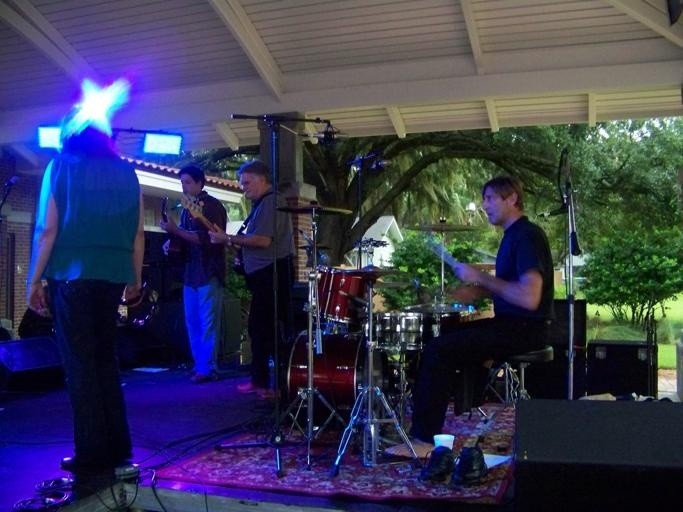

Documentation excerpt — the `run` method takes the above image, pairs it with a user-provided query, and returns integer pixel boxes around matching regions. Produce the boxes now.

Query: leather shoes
[61,455,77,470]
[235,382,280,399]
[185,370,217,384]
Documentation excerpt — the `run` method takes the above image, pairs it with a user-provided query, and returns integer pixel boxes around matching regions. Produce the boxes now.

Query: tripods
[216,106,321,477]
[288,268,421,475]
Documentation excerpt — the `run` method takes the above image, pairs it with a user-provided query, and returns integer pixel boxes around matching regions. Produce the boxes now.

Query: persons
[25,109,145,475]
[208,161,296,399]
[159,164,226,381]
[384,176,554,459]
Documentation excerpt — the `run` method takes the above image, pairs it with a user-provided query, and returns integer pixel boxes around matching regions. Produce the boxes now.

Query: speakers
[512,399,682,512]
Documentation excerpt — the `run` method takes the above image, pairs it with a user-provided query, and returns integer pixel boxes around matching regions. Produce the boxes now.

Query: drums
[362,312,424,352]
[287,331,383,428]
[313,265,368,326]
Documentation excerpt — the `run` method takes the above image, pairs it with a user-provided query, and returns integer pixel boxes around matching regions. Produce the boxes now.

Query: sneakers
[418,447,488,485]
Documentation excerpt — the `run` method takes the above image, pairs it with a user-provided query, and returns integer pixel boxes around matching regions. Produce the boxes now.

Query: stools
[504,344,555,408]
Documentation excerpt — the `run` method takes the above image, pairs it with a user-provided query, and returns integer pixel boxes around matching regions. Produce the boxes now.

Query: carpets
[148,401,518,507]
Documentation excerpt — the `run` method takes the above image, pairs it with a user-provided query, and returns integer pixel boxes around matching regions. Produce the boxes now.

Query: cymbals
[331,266,410,284]
[404,304,469,315]
[275,204,354,215]
[297,246,332,249]
[406,222,481,232]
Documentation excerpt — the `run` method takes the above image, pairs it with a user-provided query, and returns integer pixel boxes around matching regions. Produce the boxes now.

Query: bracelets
[25,280,42,285]
[224,234,233,246]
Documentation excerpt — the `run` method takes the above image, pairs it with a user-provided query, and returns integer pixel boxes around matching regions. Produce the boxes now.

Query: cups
[433,433,456,452]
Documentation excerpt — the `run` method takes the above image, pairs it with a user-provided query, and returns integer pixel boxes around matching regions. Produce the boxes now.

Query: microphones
[540,203,583,217]
[299,231,330,264]
[298,132,348,140]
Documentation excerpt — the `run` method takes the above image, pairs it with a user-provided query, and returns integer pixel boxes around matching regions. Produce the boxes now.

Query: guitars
[181,193,244,275]
[161,195,184,273]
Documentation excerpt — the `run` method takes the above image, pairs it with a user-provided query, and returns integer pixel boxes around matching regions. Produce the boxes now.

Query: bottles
[267,355,275,388]
